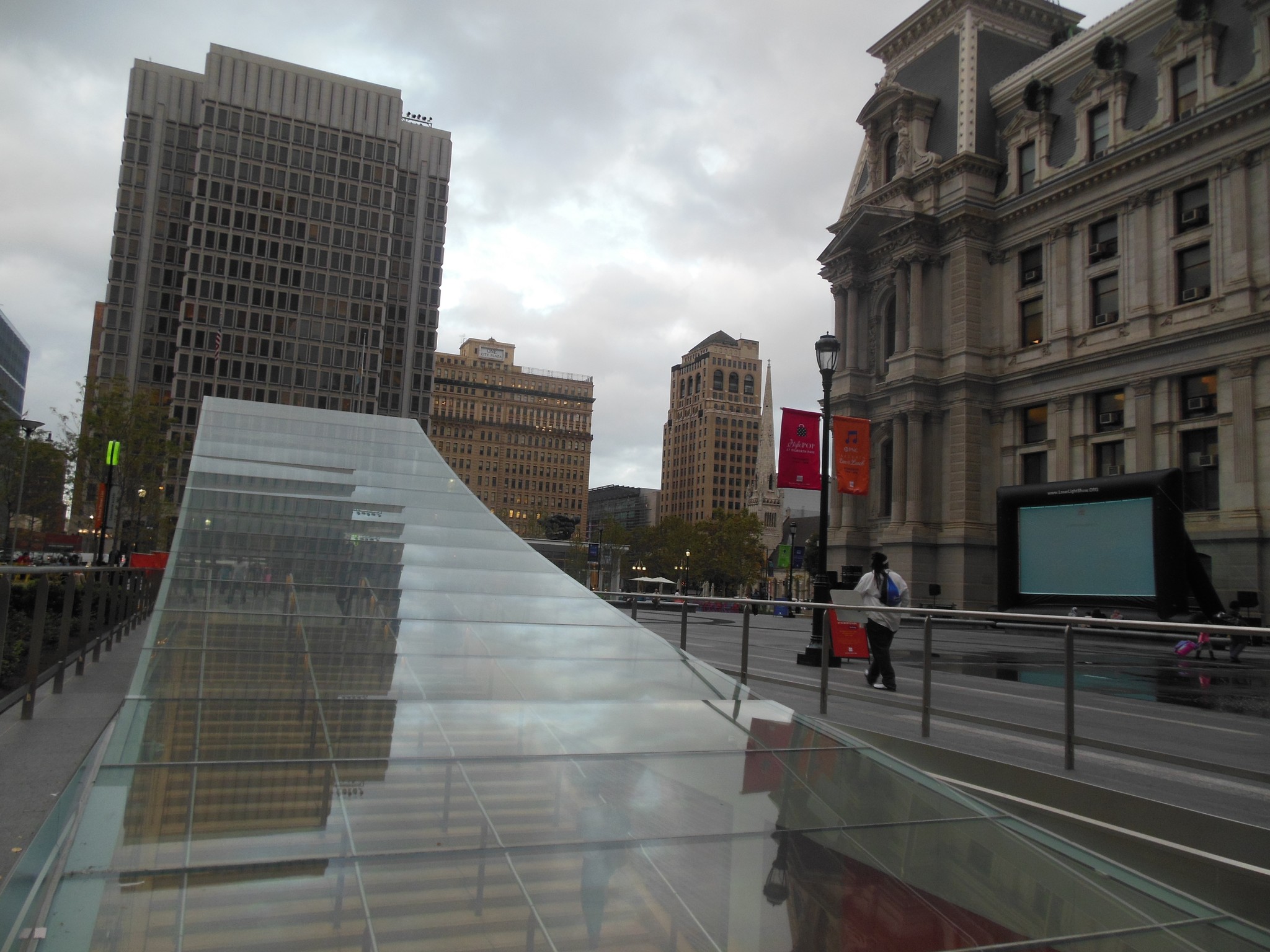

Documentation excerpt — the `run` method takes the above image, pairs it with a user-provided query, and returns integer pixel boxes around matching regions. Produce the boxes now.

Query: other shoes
[864,669,872,685]
[873,683,891,691]
[1231,658,1240,663]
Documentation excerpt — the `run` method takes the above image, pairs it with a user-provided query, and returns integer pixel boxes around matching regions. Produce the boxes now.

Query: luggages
[1173,640,1203,657]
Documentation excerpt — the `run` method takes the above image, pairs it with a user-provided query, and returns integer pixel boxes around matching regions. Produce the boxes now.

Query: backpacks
[875,571,901,606]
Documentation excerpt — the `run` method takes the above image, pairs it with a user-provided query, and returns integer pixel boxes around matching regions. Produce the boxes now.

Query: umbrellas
[629,577,653,594]
[703,581,715,598]
[646,577,678,595]
[739,584,743,599]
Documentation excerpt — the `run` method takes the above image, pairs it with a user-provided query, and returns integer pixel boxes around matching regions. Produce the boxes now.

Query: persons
[794,598,801,614]
[1067,601,1250,663]
[750,589,760,616]
[853,551,910,692]
[589,584,680,611]
[227,556,248,605]
[334,565,356,625]
[17,546,122,585]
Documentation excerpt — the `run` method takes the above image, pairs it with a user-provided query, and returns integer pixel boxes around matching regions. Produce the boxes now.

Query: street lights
[794,329,844,666]
[632,558,647,593]
[133,485,147,552]
[8,418,46,567]
[758,548,777,599]
[674,558,689,596]
[782,522,796,618]
[684,549,691,597]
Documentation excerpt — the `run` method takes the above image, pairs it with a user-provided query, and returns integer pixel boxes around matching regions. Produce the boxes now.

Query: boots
[1209,652,1218,660]
[1195,651,1202,659]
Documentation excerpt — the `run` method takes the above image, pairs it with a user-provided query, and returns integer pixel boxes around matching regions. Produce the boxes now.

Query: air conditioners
[1198,454,1218,466]
[1088,243,1106,256]
[1026,271,1041,280]
[1096,314,1114,326]
[1100,413,1118,424]
[1182,209,1204,223]
[1108,465,1124,476]
[1183,288,1205,301]
[1188,397,1209,410]
